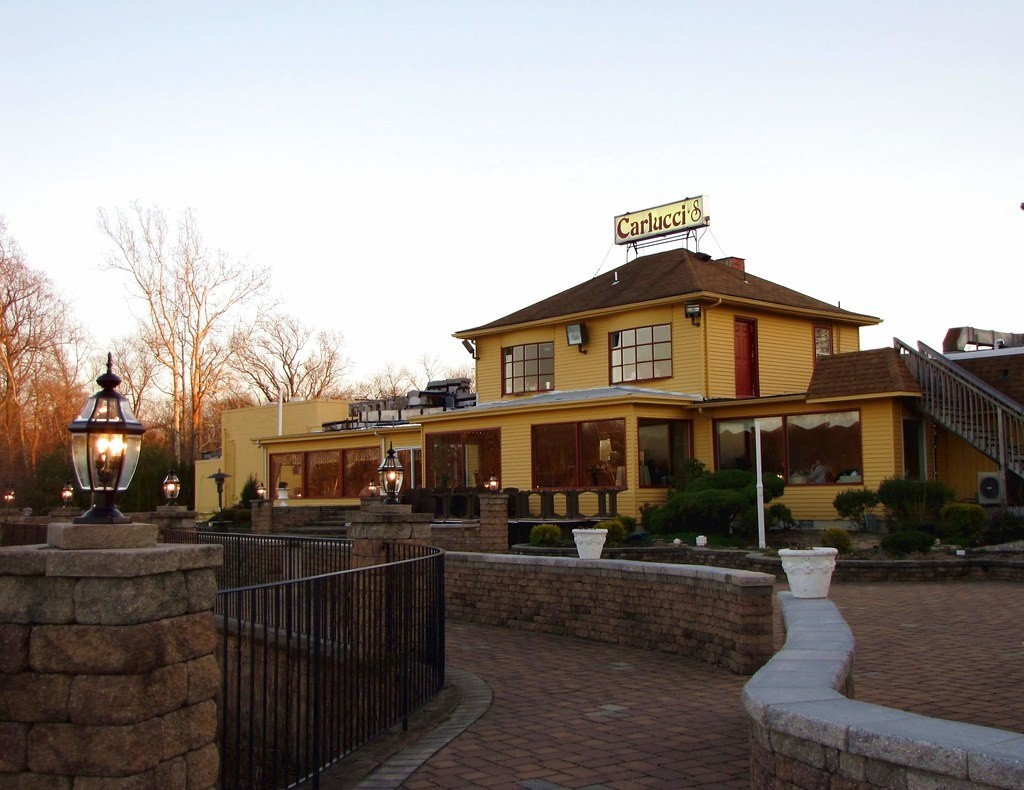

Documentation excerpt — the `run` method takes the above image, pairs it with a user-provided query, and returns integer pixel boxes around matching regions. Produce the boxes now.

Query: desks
[790,476,812,483]
[839,474,861,482]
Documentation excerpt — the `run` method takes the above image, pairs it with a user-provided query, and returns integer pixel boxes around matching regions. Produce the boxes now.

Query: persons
[735,456,747,470]
[810,459,826,484]
[835,468,862,482]
[823,462,834,480]
[565,459,573,480]
[591,466,599,485]
[778,457,784,474]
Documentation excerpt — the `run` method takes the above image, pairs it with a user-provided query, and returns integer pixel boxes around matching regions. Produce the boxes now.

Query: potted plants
[778,544,838,599]
[276,481,289,507]
[571,527,608,560]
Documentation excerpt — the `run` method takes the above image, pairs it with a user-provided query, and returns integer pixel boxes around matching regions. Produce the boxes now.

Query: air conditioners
[978,473,1001,504]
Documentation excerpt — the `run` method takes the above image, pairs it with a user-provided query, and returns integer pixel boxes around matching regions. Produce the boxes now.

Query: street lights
[206,466,232,513]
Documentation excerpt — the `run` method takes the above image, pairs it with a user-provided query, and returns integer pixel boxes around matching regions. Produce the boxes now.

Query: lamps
[206,468,231,492]
[488,473,500,493]
[61,480,75,508]
[462,339,479,360]
[368,478,377,497]
[377,440,404,503]
[68,352,146,523]
[566,323,588,354]
[684,302,701,326]
[162,467,181,506]
